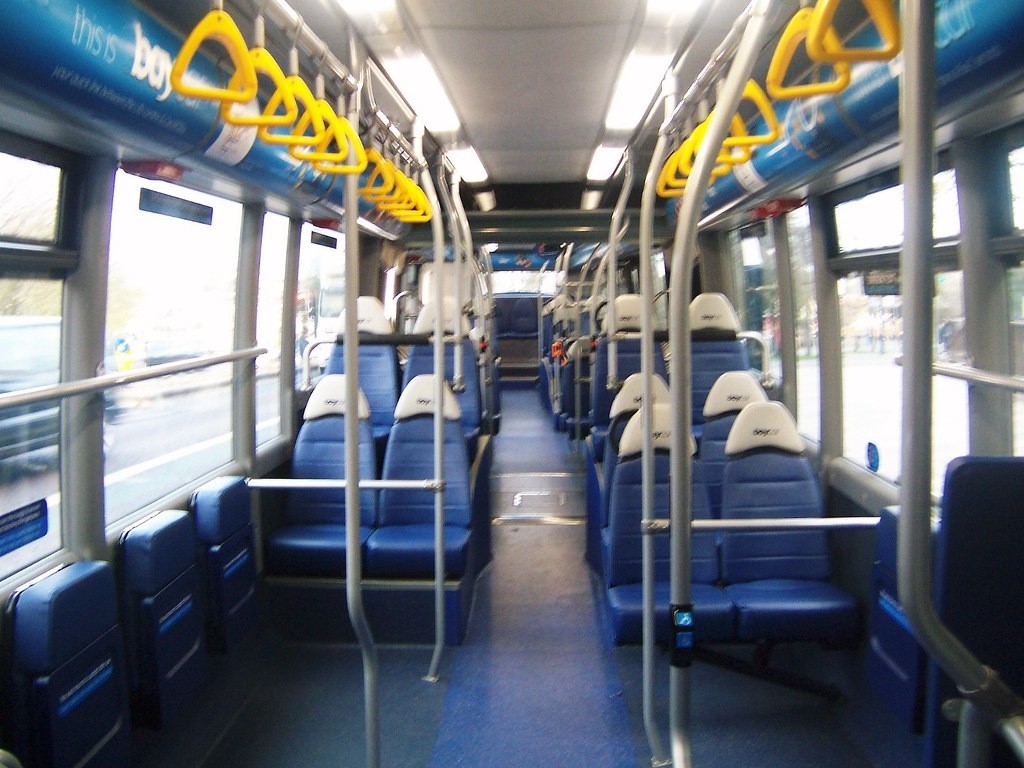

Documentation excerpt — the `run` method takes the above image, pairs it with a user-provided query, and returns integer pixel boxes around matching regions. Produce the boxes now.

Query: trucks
[316,277,345,374]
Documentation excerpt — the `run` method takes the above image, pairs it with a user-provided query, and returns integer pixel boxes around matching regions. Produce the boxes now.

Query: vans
[0,315,120,468]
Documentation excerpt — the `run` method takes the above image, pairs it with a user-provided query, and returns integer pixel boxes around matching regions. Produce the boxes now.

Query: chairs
[2,559,130,768]
[589,293,670,462]
[326,295,397,442]
[189,476,258,656]
[720,402,859,641]
[365,374,473,581]
[689,291,749,437]
[264,374,377,578]
[605,406,735,648]
[924,456,1024,768]
[700,371,769,546]
[117,510,208,731]
[606,373,696,529]
[401,296,482,445]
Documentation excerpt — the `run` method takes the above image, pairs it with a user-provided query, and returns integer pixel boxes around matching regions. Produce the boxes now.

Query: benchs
[494,298,545,341]
